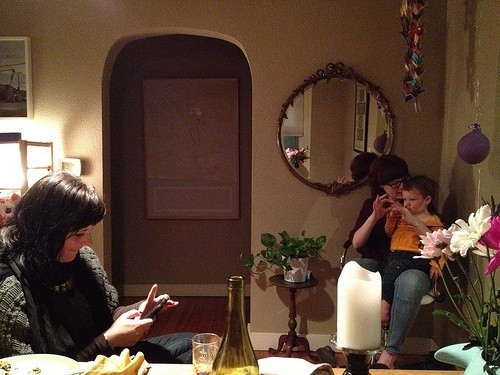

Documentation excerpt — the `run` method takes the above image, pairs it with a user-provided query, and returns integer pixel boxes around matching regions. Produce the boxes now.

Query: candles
[336,260,382,350]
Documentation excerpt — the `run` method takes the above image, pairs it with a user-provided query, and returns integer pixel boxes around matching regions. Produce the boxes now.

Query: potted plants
[240,230,327,282]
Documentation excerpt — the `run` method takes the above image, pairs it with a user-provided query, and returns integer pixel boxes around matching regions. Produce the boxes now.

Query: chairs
[340,241,444,369]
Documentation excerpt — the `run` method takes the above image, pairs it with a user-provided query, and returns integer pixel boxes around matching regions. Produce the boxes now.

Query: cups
[191,333,221,375]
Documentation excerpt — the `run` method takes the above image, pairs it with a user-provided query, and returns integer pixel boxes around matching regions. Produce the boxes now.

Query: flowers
[414,196,500,372]
[285,144,309,169]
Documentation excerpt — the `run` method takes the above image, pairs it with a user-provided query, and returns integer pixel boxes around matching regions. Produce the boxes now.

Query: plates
[0,354,80,375]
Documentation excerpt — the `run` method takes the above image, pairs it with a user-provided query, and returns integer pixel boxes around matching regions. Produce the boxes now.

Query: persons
[0,170,208,365]
[380,174,447,322]
[336,152,378,185]
[343,155,434,369]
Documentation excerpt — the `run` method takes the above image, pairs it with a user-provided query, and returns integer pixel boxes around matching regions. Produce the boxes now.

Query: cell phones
[145,298,166,318]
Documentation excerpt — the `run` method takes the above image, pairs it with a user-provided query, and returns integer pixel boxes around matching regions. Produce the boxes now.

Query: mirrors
[277,61,396,196]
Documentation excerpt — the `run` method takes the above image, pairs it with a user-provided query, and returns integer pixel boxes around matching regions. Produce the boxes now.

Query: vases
[434,343,500,375]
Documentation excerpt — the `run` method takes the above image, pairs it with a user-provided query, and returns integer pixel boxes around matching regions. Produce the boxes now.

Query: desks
[267,275,320,361]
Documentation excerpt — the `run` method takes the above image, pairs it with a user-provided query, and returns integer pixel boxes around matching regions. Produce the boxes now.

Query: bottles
[211,275,260,375]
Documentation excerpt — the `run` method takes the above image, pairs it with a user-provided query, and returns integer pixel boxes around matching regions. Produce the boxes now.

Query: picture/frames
[0,36,34,120]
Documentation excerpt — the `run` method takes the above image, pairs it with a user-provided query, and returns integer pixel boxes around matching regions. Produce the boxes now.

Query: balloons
[374,133,387,153]
[456,127,491,166]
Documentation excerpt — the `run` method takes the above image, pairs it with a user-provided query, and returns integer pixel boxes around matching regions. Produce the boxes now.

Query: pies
[79,349,144,375]
